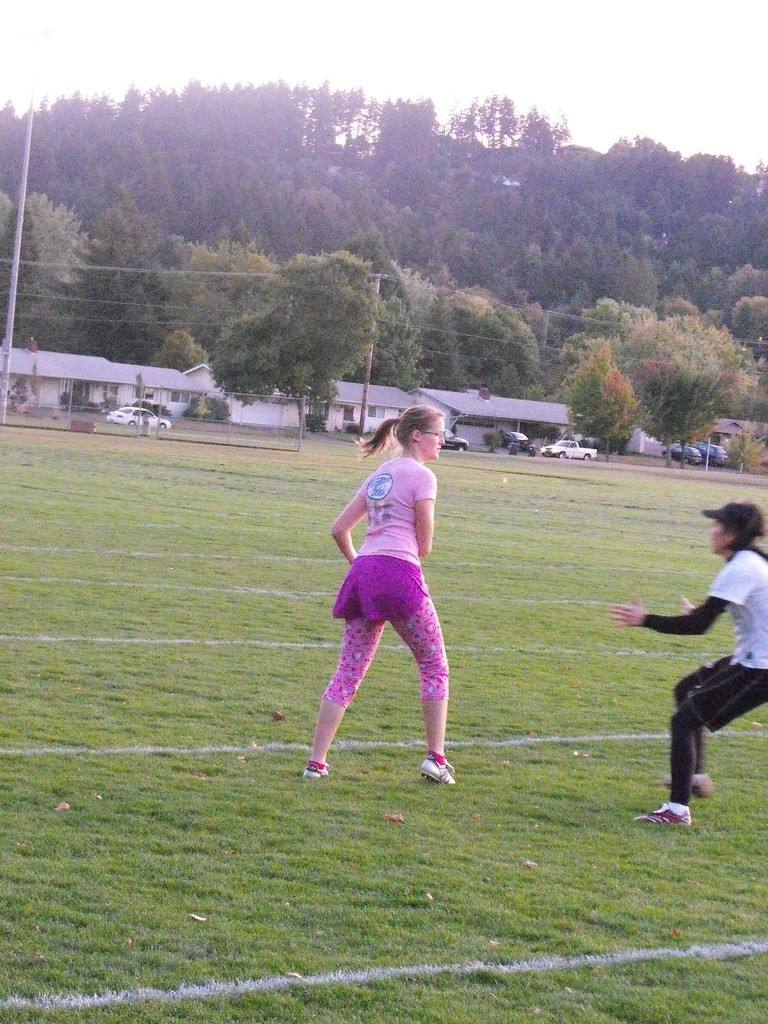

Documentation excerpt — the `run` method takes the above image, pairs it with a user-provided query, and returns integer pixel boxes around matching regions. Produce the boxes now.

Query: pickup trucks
[541,440,598,461]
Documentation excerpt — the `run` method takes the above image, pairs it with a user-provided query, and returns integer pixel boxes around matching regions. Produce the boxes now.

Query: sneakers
[632,803,694,826]
[661,773,720,798]
[417,751,456,786]
[303,763,329,779]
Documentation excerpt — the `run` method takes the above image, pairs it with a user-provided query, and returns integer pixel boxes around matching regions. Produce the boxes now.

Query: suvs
[662,447,702,465]
[503,431,531,452]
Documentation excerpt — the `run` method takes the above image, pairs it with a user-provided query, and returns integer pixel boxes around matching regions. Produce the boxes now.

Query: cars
[440,429,469,451]
[106,407,171,429]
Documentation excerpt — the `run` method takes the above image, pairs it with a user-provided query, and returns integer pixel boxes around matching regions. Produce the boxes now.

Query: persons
[607,502,768,823]
[303,406,455,785]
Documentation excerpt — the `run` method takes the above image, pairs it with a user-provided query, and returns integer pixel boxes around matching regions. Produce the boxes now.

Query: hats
[700,498,765,539]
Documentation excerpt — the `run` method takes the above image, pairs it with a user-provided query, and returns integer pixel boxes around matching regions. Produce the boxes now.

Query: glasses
[422,430,448,439]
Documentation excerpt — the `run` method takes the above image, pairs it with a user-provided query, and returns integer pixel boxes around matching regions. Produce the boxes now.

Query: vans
[696,442,728,467]
